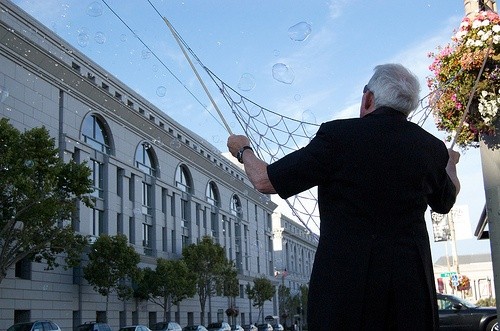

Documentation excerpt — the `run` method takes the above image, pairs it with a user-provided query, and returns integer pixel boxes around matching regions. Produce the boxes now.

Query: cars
[118,319,285,331]
[75,320,112,331]
[437,293,499,331]
[6,319,61,331]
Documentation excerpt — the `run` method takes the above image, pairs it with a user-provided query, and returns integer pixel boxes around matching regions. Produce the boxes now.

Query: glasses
[363,85,374,96]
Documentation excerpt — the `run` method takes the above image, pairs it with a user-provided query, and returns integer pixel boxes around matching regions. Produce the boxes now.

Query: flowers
[424,10,500,151]
[449,274,470,290]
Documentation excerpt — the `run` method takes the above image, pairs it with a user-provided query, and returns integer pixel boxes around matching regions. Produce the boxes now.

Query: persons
[228,63,461,331]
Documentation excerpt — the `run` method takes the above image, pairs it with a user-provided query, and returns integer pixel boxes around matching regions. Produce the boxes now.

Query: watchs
[237,146,254,164]
